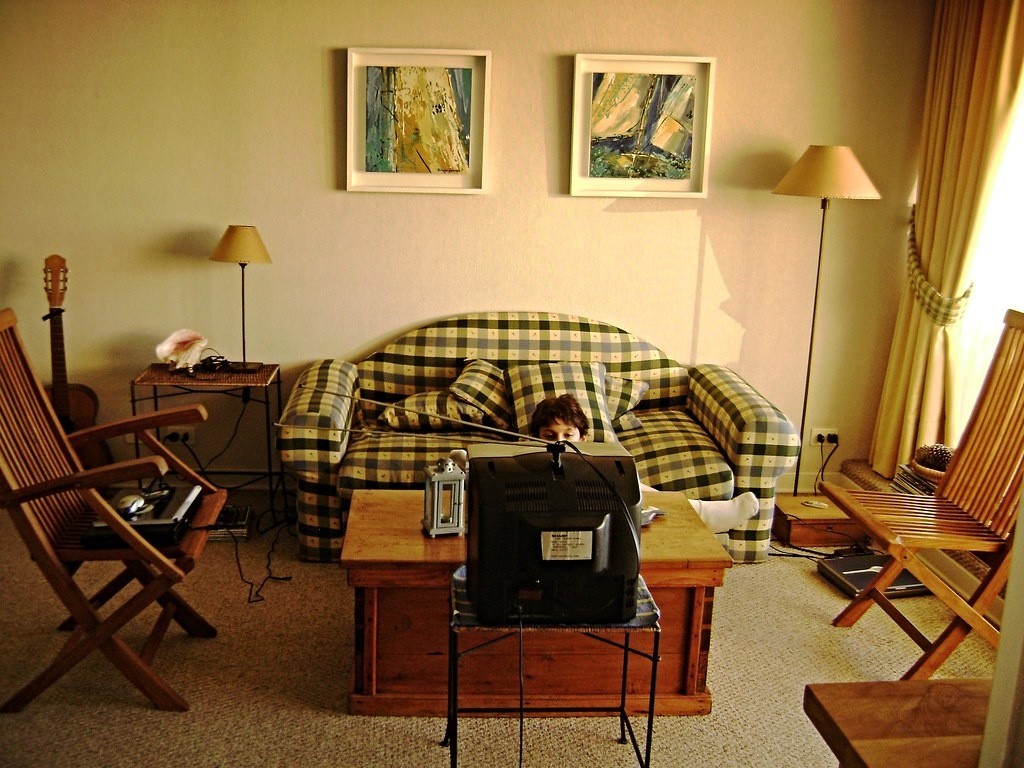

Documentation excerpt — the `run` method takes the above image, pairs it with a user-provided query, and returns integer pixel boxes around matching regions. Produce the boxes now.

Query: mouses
[117,494,144,521]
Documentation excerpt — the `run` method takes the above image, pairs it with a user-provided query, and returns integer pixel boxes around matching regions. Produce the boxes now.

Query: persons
[529,392,760,534]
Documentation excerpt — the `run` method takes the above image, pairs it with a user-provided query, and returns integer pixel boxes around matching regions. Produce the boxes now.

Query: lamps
[208,225,273,373]
[771,143,884,498]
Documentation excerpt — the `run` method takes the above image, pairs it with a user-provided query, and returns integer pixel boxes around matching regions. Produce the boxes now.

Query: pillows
[604,373,651,432]
[377,390,467,430]
[450,357,520,439]
[502,362,621,444]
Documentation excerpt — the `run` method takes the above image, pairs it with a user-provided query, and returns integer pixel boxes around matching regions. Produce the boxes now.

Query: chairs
[832,309,1024,681]
[0,308,228,715]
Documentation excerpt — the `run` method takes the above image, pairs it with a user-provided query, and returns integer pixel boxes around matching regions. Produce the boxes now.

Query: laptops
[82,484,205,550]
[817,554,933,600]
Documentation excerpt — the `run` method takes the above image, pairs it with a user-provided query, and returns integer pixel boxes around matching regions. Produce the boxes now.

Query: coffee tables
[340,489,735,716]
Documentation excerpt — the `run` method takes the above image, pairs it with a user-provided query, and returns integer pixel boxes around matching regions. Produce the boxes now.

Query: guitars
[40,254,115,499]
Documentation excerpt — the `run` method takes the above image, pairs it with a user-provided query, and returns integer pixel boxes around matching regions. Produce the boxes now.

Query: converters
[834,547,874,557]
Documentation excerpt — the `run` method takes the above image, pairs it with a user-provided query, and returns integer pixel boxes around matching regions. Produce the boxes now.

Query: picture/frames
[571,53,716,199]
[347,45,495,197]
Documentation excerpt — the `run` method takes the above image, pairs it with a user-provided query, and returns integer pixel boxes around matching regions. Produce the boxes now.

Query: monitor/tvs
[273,383,643,628]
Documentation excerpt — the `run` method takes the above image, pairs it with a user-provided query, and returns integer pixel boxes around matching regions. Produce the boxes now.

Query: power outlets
[164,425,196,446]
[810,428,839,446]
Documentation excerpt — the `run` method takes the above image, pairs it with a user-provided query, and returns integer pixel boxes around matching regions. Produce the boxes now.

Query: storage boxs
[773,492,873,545]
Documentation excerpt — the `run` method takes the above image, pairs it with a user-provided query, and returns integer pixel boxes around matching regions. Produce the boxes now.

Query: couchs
[276,311,802,566]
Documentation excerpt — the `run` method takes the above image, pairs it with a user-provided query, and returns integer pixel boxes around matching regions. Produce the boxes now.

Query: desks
[442,566,662,768]
[130,361,283,533]
[803,677,1024,767]
